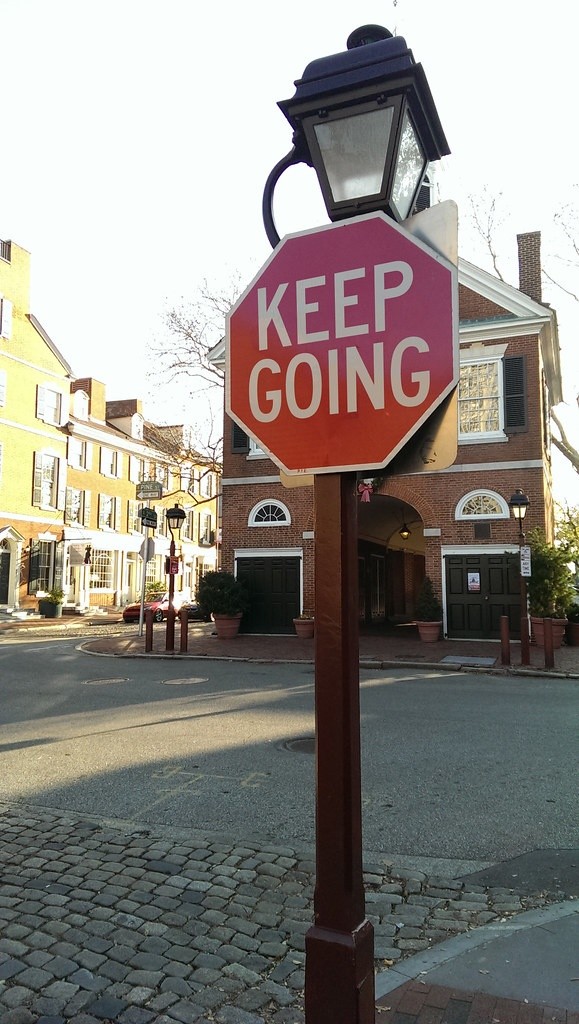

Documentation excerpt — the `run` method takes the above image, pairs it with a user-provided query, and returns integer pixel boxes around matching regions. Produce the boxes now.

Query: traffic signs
[136,481,162,500]
[140,506,157,528]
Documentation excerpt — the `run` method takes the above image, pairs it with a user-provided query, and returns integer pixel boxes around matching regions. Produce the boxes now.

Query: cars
[122,592,182,623]
[178,598,215,623]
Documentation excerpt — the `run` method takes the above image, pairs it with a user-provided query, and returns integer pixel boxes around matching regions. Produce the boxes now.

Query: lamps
[399,508,411,540]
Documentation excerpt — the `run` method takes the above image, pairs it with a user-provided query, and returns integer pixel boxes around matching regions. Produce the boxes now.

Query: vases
[293,616,314,639]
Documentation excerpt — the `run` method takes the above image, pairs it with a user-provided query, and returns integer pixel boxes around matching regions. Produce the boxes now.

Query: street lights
[508,488,531,665]
[263,22,450,1024]
[165,503,187,650]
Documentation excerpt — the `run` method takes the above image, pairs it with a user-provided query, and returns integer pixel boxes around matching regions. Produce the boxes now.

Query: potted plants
[195,569,245,641]
[504,526,578,651]
[38,586,64,618]
[565,601,579,646]
[415,576,443,643]
